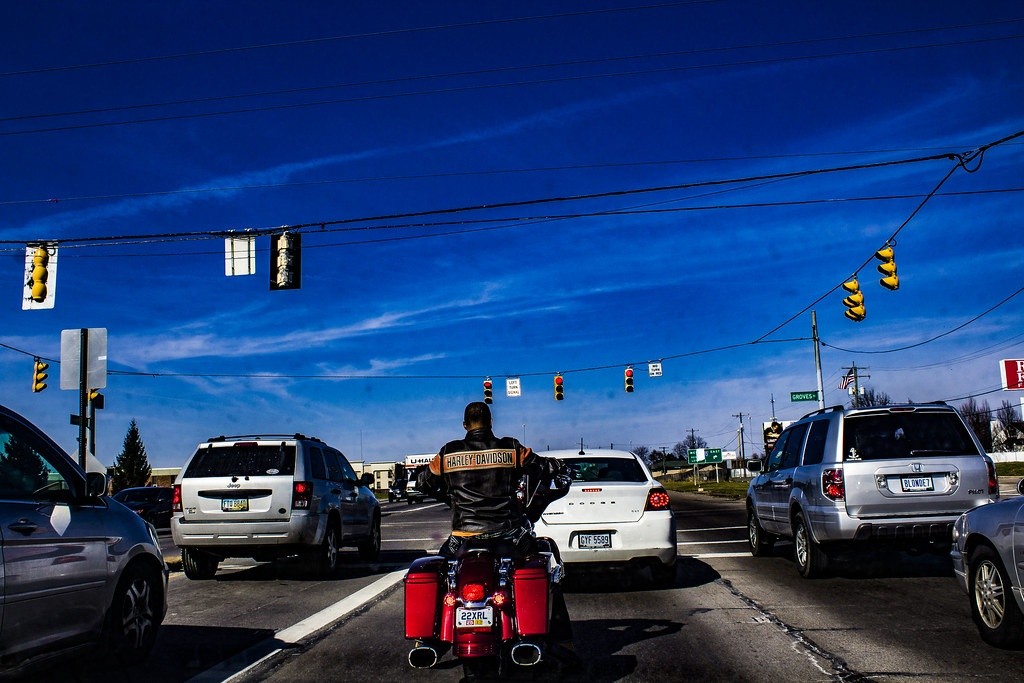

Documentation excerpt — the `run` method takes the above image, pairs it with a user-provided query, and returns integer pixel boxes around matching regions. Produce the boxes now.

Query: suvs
[746,401,1003,580]
[169,432,382,580]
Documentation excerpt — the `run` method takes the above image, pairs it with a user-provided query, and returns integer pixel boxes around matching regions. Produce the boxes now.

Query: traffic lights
[483,380,493,405]
[553,376,564,400]
[876,246,900,291]
[842,279,866,322]
[625,368,634,393]
[88,388,100,401]
[31,361,49,393]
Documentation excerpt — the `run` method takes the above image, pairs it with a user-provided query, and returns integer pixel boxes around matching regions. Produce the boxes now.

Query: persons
[416,401,550,562]
[764,422,783,443]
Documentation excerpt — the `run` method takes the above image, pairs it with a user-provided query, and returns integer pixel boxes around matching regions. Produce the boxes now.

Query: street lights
[521,424,526,446]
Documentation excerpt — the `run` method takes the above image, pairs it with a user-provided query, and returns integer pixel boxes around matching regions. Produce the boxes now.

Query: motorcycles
[402,536,554,683]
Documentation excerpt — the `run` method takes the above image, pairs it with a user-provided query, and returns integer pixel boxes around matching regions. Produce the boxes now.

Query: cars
[950,478,1024,650]
[388,479,407,505]
[109,487,174,529]
[0,404,170,683]
[533,448,677,589]
[405,473,428,505]
